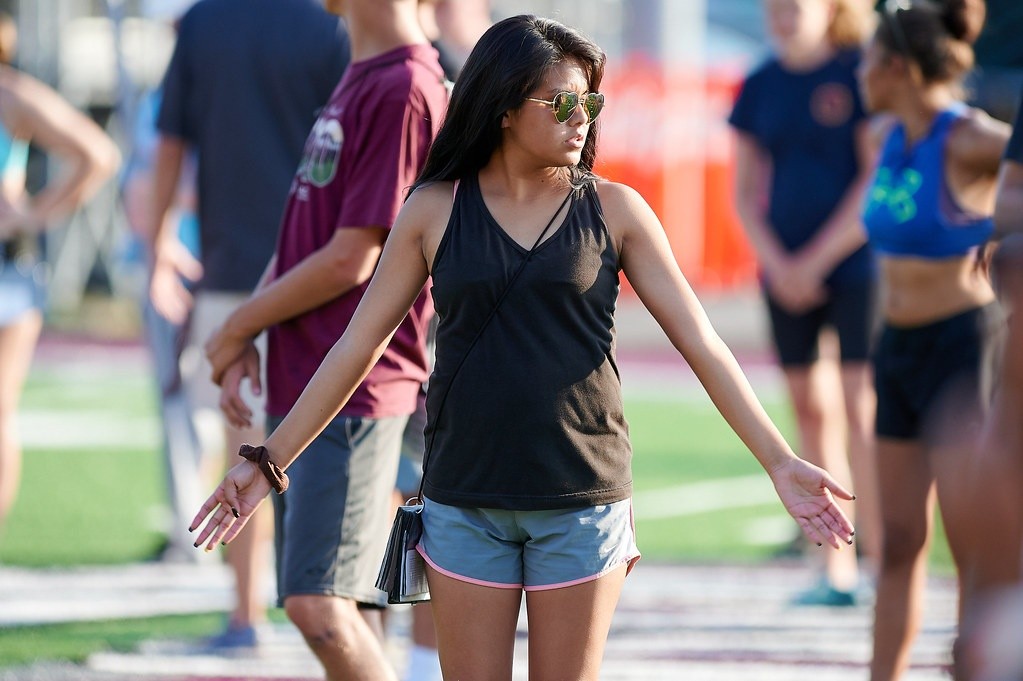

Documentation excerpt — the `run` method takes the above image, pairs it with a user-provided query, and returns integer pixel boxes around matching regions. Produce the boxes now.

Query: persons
[767,0,1023,681]
[724,0,880,602]
[142,0,351,652]
[189,15,856,681]
[204,0,451,681]
[0,0,503,657]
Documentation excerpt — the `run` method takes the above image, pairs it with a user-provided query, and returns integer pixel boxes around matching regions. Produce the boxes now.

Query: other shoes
[194,623,276,658]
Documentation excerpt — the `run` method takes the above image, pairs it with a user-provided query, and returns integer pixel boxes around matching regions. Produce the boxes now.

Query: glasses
[874,0,909,49]
[522,91,604,125]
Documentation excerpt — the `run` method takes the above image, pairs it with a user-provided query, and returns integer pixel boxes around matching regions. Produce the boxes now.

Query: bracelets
[237,441,290,495]
